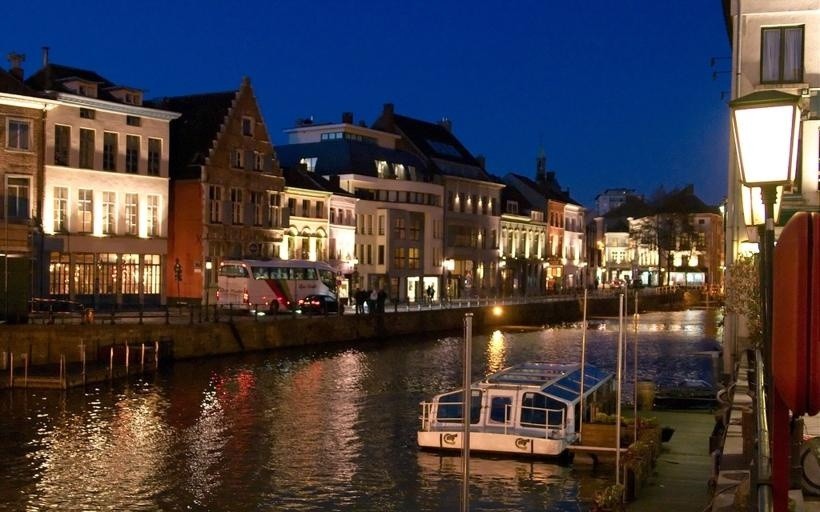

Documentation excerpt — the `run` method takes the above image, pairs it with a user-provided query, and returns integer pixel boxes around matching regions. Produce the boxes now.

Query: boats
[416,358,618,462]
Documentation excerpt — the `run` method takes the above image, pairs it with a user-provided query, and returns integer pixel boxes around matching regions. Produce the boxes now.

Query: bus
[218,260,341,314]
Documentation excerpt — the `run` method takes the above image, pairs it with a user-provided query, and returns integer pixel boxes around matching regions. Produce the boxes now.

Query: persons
[427,284,431,297]
[429,285,435,300]
[594,278,599,290]
[353,285,388,315]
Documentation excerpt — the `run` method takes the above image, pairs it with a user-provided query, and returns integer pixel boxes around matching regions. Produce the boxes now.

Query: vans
[25,298,86,326]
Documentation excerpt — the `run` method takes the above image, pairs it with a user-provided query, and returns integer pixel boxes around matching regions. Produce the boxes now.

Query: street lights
[443,258,455,305]
[737,178,784,345]
[203,255,211,322]
[725,91,803,510]
[720,265,726,278]
[600,266,608,294]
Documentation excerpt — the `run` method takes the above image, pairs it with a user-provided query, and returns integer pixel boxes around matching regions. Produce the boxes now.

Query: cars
[302,294,345,313]
